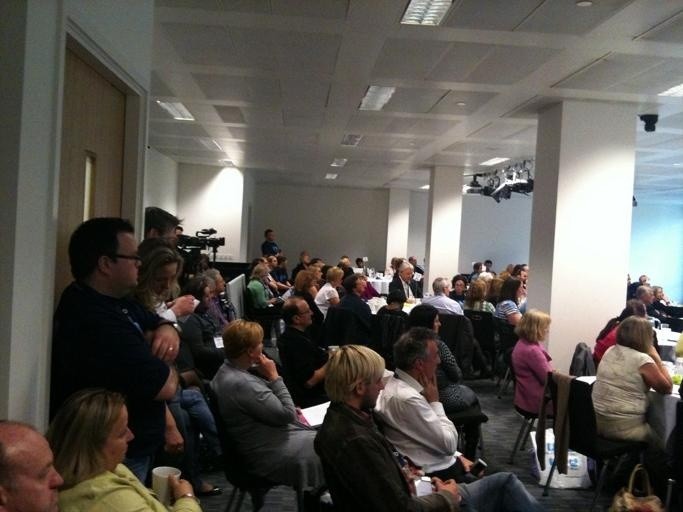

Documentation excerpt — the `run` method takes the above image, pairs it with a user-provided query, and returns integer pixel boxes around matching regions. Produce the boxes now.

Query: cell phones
[469,458,488,476]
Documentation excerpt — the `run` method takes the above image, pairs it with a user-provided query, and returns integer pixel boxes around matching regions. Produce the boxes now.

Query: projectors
[463,184,484,196]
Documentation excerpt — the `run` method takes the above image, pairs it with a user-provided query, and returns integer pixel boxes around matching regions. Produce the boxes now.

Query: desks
[646,380,679,476]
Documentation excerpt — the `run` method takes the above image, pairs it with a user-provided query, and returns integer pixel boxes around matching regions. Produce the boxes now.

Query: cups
[151,465,180,502]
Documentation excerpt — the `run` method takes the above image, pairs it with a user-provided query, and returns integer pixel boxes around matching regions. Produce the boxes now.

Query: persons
[592,274,682,511]
[1,205,547,512]
[510,309,556,418]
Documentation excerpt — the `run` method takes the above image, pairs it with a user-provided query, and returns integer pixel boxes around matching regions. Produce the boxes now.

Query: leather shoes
[196,485,224,497]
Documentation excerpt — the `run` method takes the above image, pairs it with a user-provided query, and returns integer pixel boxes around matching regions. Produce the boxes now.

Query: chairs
[542,370,648,509]
[505,348,556,467]
[570,343,595,374]
[628,281,682,357]
[211,265,505,504]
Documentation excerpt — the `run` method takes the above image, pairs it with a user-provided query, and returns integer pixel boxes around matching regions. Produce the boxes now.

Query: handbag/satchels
[606,463,667,512]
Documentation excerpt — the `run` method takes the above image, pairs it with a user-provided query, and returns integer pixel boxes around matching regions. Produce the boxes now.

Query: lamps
[459,159,536,203]
